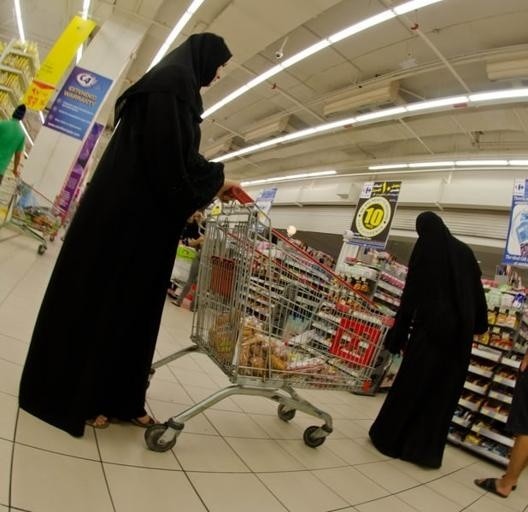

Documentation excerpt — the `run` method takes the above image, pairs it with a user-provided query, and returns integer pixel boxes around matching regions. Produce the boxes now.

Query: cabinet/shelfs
[447,282,528,470]
[0,38,39,122]
[319,243,407,396]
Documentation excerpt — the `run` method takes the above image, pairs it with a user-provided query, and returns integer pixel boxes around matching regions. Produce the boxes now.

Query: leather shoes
[167,291,197,313]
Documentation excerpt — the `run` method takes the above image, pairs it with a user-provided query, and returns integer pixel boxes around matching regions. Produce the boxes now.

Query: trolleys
[139,186,392,458]
[0,176,68,256]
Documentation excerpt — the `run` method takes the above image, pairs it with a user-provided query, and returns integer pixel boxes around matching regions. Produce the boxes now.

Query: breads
[239,328,288,378]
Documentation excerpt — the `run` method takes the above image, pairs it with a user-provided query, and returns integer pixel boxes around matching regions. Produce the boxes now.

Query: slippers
[474,477,511,498]
[501,473,517,491]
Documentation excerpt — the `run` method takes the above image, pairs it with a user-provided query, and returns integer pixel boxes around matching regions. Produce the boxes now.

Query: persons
[471,346,528,500]
[169,209,212,311]
[1,103,27,180]
[16,33,244,438]
[366,212,488,470]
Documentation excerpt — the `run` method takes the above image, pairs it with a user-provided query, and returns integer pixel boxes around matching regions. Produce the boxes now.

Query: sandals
[82,410,110,429]
[129,411,159,428]
